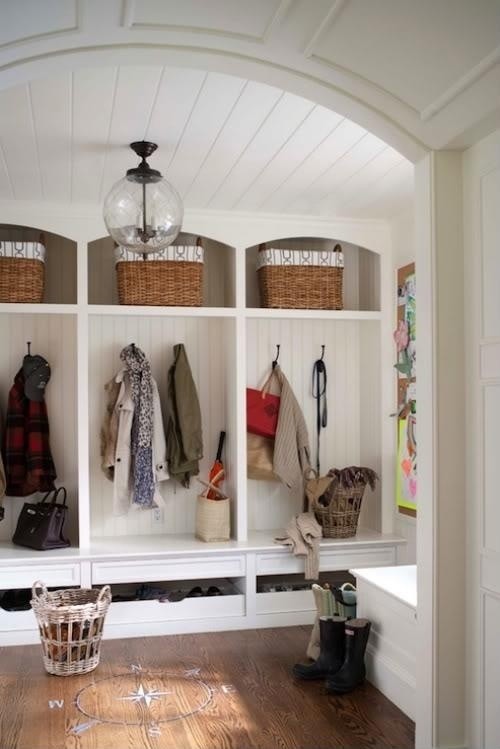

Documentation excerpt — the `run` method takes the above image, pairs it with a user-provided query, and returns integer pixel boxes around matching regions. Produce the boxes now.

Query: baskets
[258,239,345,310]
[0,232,48,303]
[303,466,366,539]
[112,237,205,309]
[32,579,114,677]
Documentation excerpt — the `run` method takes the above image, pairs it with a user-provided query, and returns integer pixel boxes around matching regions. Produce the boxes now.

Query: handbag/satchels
[306,581,358,661]
[193,470,233,544]
[247,368,284,481]
[12,487,72,551]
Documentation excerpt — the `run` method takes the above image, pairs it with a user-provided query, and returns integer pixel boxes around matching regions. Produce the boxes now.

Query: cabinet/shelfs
[0,201,414,649]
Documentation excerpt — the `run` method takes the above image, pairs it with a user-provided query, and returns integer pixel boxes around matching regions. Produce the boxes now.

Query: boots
[292,615,348,680]
[321,617,371,695]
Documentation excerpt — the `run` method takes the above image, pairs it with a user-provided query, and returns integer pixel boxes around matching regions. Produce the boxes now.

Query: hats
[20,353,52,402]
